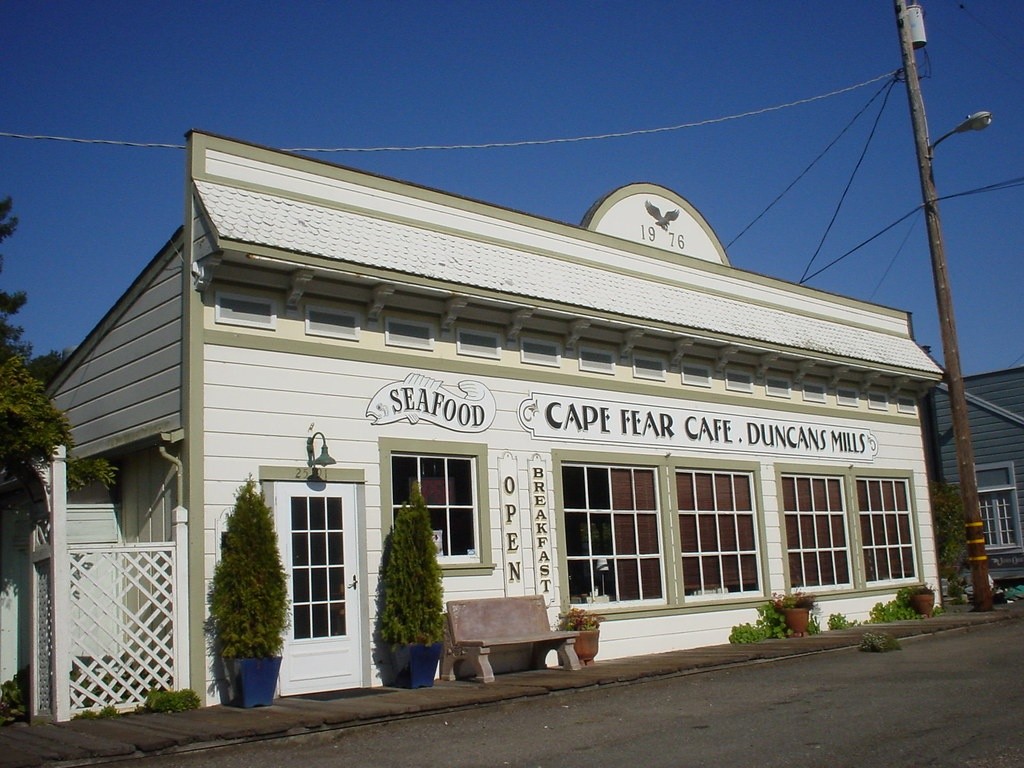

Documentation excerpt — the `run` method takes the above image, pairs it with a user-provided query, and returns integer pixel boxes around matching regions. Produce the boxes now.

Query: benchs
[444,595,582,683]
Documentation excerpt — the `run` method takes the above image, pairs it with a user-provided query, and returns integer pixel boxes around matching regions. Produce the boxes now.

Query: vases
[785,608,809,632]
[574,631,600,661]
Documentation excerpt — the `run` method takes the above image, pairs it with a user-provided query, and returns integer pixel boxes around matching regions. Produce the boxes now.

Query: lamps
[307,431,337,467]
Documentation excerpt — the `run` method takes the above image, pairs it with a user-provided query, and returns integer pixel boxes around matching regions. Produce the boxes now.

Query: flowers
[554,607,606,632]
[770,587,817,609]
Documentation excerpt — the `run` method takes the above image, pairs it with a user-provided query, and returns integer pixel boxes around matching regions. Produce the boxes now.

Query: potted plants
[201,470,292,709]
[380,479,447,688]
[943,545,967,573]
[911,584,939,613]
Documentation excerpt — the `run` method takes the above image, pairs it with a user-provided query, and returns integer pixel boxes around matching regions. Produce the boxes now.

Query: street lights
[904,112,994,612]
